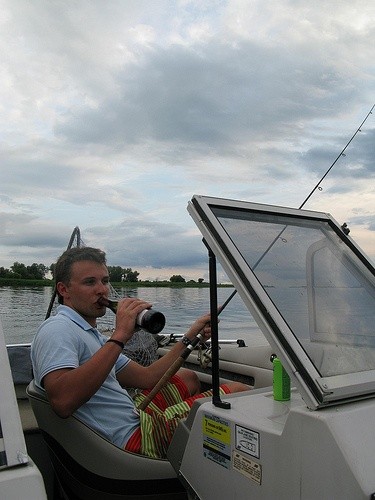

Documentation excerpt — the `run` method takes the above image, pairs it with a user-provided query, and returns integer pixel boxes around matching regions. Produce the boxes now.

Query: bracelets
[105,339,125,350]
[181,335,191,345]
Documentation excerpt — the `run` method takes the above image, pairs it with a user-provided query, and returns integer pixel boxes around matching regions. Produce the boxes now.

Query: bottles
[97,296,166,336]
[273,355,291,402]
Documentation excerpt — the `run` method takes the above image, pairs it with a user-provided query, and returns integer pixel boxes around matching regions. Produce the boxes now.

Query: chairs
[23,375,180,480]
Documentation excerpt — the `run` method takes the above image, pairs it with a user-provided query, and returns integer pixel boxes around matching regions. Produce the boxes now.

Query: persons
[30,247,254,459]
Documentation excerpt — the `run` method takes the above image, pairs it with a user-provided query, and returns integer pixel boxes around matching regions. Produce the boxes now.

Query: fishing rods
[136,103,375,412]
